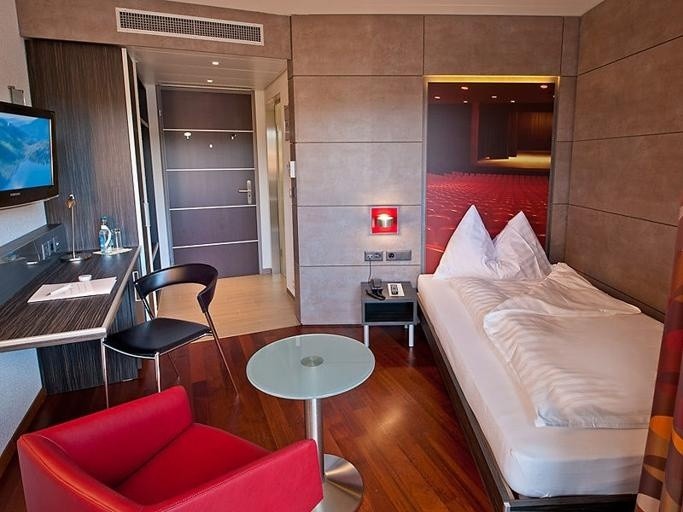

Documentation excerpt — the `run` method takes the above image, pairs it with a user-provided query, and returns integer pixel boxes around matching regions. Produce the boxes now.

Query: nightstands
[361,281,417,348]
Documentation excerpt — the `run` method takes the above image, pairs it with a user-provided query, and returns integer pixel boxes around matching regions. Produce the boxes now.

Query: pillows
[432,203,552,284]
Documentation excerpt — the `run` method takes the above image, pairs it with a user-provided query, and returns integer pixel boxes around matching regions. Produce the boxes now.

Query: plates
[94,249,131,255]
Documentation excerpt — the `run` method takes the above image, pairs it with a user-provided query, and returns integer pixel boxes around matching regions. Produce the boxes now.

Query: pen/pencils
[46,287,64,296]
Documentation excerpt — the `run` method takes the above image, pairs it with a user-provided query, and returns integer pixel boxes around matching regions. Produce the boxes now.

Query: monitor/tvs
[0,101,61,210]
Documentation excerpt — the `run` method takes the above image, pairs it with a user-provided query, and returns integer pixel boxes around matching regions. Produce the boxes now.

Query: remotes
[391,284,399,295]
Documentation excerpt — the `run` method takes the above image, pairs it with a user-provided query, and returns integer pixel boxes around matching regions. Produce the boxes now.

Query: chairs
[100,262,238,409]
[15,383,323,512]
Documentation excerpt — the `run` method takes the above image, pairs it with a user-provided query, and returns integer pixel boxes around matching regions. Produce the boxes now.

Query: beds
[418,263,665,511]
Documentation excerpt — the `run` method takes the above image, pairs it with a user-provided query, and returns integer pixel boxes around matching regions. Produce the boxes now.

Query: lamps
[60,195,92,263]
[370,206,398,234]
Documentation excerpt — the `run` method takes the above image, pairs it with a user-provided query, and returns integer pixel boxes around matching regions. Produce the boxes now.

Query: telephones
[371,278,383,291]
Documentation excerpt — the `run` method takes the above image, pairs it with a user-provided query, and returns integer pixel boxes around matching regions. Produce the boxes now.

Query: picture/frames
[421,74,562,273]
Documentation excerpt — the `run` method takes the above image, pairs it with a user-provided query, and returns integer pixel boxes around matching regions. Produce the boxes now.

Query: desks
[1,245,140,409]
[243,333,377,512]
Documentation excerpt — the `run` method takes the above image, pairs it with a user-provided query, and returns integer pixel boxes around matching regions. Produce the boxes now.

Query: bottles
[110,228,123,250]
[99,213,112,252]
